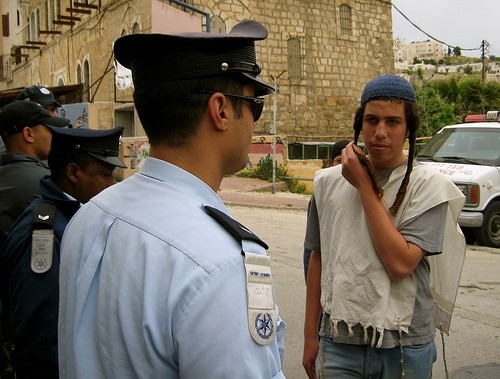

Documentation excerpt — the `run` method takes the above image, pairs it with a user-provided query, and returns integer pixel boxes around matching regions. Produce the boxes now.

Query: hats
[0,100,70,134]
[16,84,61,108]
[46,122,128,170]
[360,73,417,104]
[113,19,276,97]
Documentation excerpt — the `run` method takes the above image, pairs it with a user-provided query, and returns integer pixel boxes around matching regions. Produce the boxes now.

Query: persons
[0,100,71,251]
[57,21,287,379]
[16,86,62,116]
[304,140,351,285]
[0,126,126,379]
[302,75,467,379]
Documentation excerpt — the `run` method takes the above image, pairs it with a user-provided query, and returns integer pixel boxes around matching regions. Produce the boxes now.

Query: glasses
[204,89,265,122]
[333,156,342,166]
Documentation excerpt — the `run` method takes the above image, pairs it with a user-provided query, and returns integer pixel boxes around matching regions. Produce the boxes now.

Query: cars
[412,111,500,247]
